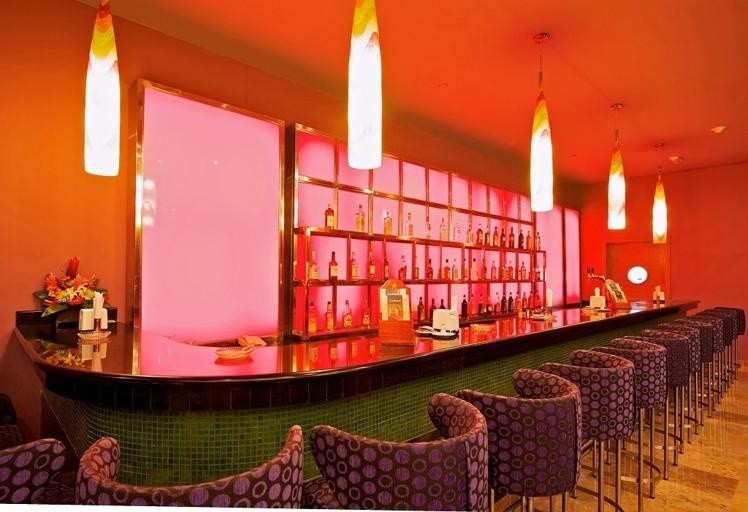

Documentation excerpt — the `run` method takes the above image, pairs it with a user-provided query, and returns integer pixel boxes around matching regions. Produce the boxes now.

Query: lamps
[527,28,670,246]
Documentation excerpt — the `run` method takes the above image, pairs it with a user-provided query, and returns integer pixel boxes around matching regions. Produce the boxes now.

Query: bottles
[95,318,101,332]
[405,212,414,236]
[383,210,392,235]
[308,250,319,279]
[326,203,335,229]
[467,223,543,251]
[438,216,448,239]
[329,250,338,279]
[367,254,542,282]
[350,251,360,279]
[356,204,365,232]
[657,295,660,306]
[308,300,371,330]
[416,291,543,324]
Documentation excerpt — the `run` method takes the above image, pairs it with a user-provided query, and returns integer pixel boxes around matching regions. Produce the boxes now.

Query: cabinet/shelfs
[287,120,537,343]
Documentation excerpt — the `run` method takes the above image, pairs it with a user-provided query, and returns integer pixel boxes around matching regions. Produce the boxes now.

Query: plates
[76,331,111,340]
[416,330,438,336]
[214,347,253,359]
[432,332,454,338]
[529,314,547,320]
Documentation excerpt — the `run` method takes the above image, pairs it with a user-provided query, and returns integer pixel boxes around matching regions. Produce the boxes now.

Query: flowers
[31,255,112,318]
